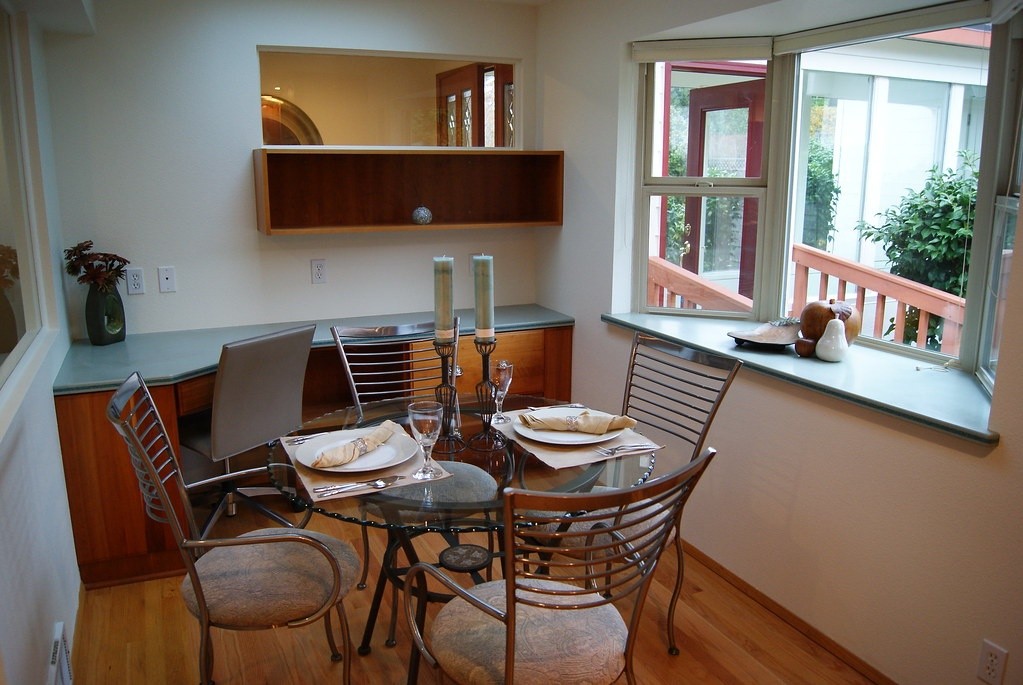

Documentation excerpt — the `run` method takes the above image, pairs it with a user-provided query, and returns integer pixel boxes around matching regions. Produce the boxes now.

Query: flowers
[56,238,132,290]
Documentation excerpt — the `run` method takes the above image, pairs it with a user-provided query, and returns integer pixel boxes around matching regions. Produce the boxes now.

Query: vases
[87,285,128,347]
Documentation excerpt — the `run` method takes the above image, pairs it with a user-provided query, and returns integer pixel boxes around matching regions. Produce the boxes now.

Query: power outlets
[125,268,148,297]
[311,259,325,284]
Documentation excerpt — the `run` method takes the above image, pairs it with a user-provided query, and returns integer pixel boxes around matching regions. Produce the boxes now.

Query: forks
[595,445,657,457]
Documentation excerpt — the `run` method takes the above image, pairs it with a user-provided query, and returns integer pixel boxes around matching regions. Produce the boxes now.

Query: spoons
[318,476,399,498]
[528,406,586,411]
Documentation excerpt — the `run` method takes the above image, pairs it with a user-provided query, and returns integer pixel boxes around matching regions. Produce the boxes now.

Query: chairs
[107,372,361,685]
[332,320,497,653]
[518,331,745,646]
[179,326,313,552]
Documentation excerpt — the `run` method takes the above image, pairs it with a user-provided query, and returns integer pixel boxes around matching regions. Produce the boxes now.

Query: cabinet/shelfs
[61,302,576,593]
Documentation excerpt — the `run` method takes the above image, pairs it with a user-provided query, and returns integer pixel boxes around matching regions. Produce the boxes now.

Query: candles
[433,254,456,344]
[471,253,496,343]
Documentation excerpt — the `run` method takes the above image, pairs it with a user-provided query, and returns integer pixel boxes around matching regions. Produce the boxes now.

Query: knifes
[313,476,406,493]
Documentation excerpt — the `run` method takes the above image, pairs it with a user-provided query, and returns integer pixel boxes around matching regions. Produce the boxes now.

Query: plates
[513,408,625,445]
[295,428,419,472]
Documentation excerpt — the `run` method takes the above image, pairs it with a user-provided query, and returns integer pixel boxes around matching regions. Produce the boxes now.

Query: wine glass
[489,360,513,424]
[408,401,443,480]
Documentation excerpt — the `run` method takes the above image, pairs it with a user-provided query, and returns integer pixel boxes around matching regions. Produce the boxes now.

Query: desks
[397,449,715,685]
[270,392,602,677]
